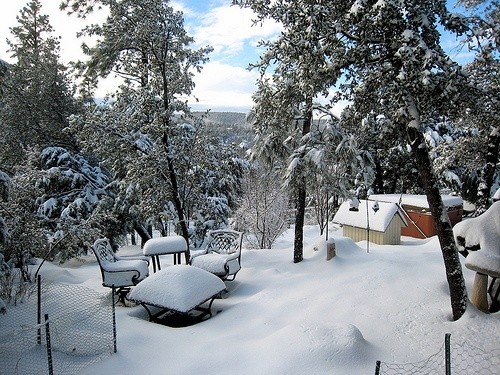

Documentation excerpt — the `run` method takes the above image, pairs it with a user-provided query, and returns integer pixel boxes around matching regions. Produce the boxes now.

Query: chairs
[189,229,245,282]
[89,236,150,307]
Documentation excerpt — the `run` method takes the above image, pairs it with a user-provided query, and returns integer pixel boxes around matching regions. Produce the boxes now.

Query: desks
[457,231,499,313]
[125,287,227,327]
[145,245,188,274]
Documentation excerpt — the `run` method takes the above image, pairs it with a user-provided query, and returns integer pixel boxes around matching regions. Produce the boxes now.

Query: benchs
[465,260,500,314]
